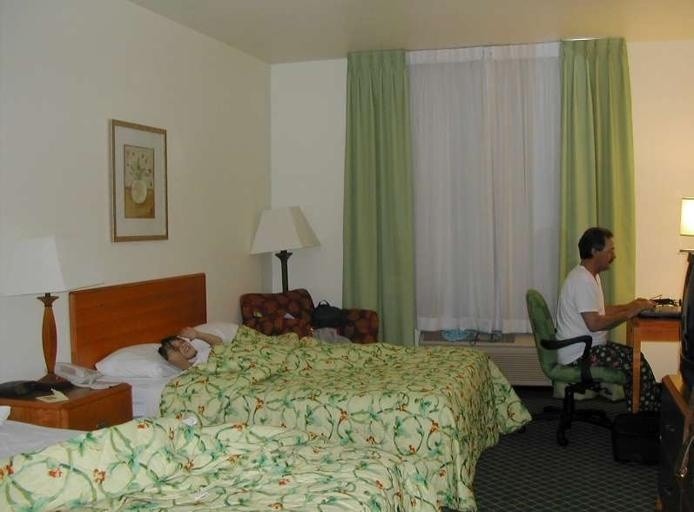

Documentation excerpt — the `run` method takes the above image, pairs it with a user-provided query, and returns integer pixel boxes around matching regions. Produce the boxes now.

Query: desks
[656,375,694,512]
[627,318,680,414]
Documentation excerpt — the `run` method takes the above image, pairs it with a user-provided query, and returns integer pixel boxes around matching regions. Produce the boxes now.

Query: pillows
[1,405,11,424]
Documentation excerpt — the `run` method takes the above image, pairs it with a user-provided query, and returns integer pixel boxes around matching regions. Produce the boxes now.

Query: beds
[0,417,456,512]
[69,273,534,511]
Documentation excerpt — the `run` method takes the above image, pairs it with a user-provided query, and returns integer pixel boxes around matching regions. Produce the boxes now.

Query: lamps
[679,197,694,237]
[5,235,105,390]
[250,206,321,292]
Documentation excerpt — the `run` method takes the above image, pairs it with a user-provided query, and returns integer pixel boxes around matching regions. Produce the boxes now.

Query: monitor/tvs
[679,254,694,386]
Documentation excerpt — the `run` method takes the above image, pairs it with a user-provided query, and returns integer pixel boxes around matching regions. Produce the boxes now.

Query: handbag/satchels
[310,299,345,330]
[610,410,661,465]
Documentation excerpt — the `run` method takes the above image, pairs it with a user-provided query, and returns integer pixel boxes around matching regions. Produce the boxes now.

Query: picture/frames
[111,119,169,241]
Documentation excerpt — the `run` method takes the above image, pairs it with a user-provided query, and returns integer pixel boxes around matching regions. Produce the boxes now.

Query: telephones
[54,362,101,384]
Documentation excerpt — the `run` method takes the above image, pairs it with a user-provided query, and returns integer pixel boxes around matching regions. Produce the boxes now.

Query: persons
[555,228,662,413]
[159,325,224,373]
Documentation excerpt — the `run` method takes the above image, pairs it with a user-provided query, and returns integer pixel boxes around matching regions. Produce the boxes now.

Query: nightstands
[1,383,133,431]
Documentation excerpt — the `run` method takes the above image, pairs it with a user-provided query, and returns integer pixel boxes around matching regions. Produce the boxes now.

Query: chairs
[241,289,378,343]
[518,290,625,447]
